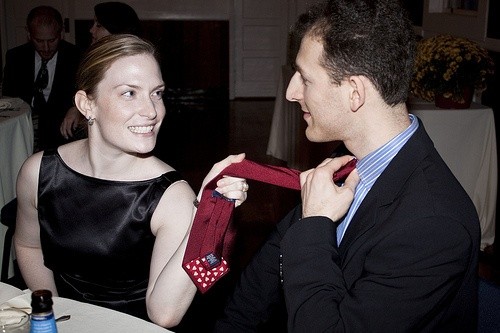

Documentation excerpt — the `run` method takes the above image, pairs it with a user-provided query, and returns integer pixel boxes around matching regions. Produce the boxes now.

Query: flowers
[408,33,494,103]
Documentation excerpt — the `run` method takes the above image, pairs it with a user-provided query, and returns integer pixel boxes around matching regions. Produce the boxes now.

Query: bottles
[29,289,58,332]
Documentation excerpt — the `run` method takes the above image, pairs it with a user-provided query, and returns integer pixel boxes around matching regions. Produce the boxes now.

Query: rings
[244,183,248,191]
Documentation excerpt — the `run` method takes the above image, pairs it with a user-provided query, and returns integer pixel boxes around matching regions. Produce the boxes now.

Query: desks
[407,104,498,253]
[0,96,35,282]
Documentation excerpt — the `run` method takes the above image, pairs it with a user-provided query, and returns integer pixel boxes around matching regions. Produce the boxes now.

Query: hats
[94,2,140,36]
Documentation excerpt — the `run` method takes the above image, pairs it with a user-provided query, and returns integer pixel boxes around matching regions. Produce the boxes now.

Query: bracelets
[192,197,201,208]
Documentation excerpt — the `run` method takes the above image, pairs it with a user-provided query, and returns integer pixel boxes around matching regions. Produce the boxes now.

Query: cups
[0,309,30,332]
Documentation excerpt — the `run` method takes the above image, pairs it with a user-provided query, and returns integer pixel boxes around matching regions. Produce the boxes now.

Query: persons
[61,1,142,140]
[1,6,86,155]
[14,32,250,327]
[182,0,482,333]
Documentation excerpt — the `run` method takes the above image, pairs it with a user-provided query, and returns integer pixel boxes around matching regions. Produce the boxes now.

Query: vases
[433,88,474,110]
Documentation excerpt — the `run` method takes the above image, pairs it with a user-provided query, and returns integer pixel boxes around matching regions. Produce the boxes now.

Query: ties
[182,158,359,294]
[32,59,49,133]
